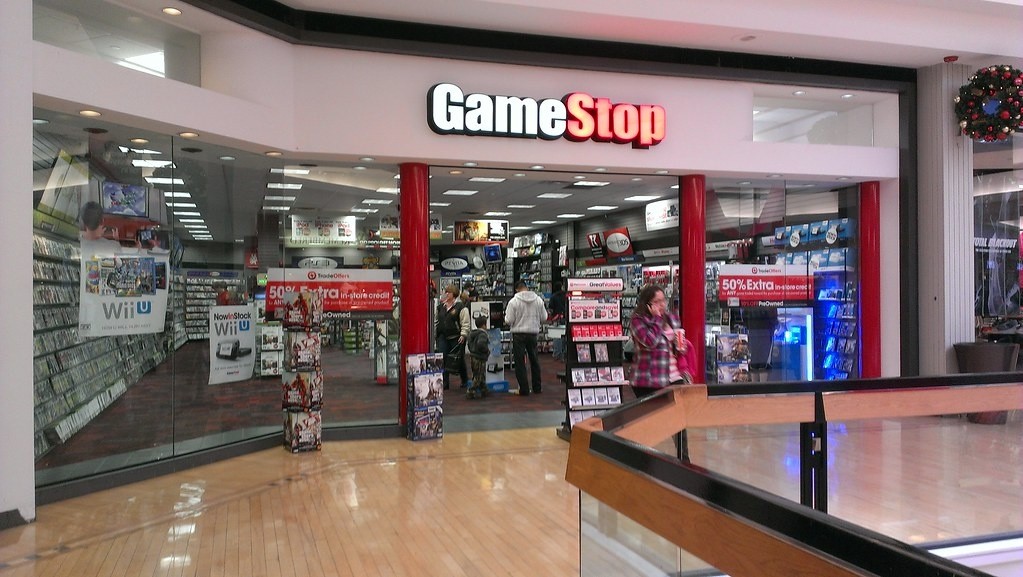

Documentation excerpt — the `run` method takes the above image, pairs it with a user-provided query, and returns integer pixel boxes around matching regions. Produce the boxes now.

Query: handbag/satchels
[445,343,464,376]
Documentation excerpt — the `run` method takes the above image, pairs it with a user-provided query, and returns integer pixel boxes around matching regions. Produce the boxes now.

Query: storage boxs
[407,349,446,442]
[254,322,283,379]
[280,328,325,452]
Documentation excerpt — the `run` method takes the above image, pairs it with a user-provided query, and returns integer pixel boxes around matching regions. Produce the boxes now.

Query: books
[594,343,609,363]
[568,366,625,428]
[576,344,591,363]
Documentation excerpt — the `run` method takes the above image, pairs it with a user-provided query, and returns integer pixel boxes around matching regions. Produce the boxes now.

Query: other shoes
[481,392,494,399]
[466,395,482,400]
[532,388,543,394]
[460,379,468,387]
[444,383,449,390]
[514,390,528,395]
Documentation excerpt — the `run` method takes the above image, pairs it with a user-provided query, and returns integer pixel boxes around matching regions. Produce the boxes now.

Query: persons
[429,276,481,314]
[212,280,231,305]
[629,286,693,464]
[466,316,496,400]
[504,280,548,396]
[80,201,122,253]
[433,284,471,391]
[743,303,782,383]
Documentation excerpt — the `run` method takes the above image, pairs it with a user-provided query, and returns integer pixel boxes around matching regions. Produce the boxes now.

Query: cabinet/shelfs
[33,226,244,466]
[620,294,638,350]
[342,321,362,354]
[813,271,859,380]
[471,301,515,368]
[506,252,563,309]
[556,277,629,442]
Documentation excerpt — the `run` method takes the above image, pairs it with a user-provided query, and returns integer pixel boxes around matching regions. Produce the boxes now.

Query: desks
[478,295,514,309]
